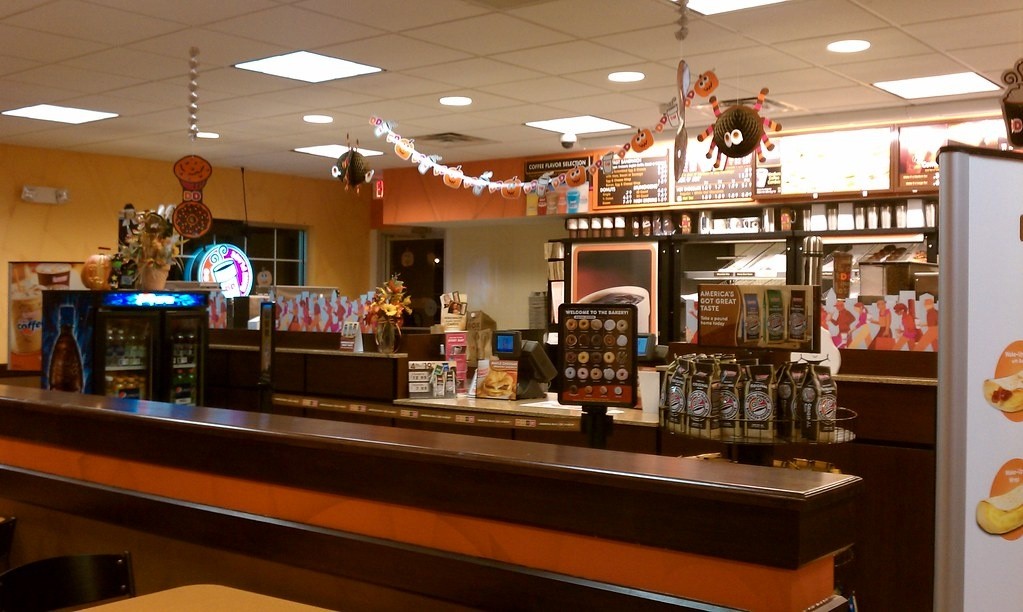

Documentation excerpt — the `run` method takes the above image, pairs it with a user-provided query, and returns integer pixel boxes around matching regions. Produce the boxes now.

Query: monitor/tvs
[637,333,656,362]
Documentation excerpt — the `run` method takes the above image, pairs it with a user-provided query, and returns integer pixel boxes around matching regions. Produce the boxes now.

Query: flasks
[801,235,823,286]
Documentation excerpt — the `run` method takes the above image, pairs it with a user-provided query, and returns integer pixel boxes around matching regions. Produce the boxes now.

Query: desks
[79,583,337,612]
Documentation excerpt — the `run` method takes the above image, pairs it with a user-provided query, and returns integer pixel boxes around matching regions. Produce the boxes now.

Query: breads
[482,372,514,396]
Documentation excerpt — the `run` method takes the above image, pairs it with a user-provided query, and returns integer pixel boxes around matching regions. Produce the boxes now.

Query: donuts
[565,319,629,380]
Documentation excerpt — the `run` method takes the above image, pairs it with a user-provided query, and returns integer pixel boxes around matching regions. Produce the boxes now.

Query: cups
[538,190,580,214]
[780,203,934,231]
[756,168,768,188]
[567,217,625,238]
[576,286,650,335]
[9,291,42,355]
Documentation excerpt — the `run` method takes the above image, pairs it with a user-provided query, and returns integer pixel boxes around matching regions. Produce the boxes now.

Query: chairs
[0,548,134,612]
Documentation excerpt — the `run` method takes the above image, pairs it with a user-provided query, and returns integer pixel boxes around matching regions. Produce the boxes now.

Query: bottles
[170,330,198,405]
[105,326,149,367]
[106,372,149,400]
[48,304,83,393]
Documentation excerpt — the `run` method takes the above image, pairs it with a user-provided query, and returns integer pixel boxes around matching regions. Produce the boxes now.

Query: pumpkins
[80,253,114,290]
